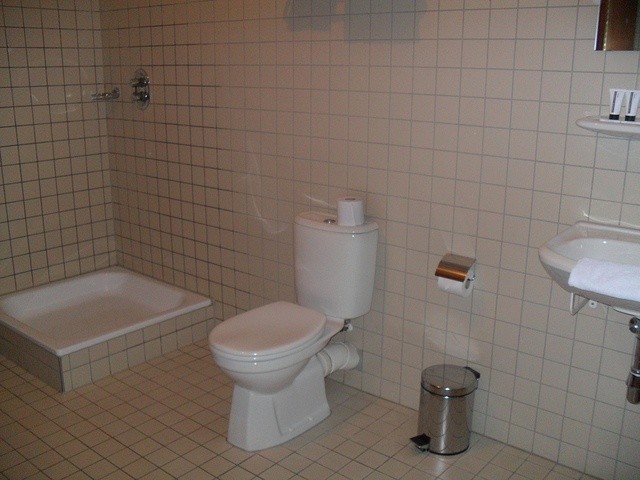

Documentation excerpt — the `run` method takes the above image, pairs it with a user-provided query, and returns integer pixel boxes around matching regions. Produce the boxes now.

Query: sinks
[571,238,640,272]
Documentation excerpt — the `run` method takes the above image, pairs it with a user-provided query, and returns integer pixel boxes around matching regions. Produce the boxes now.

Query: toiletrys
[608,88,625,121]
[624,90,640,122]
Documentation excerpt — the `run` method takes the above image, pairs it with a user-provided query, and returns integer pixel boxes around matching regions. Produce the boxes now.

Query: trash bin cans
[409,364,480,456]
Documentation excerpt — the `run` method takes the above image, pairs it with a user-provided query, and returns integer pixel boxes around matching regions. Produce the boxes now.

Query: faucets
[90,86,120,101]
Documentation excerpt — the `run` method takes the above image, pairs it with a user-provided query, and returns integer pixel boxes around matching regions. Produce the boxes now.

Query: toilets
[208,211,380,451]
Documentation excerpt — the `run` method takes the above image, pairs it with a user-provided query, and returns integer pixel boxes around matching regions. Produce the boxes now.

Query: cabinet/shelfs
[577,115,640,139]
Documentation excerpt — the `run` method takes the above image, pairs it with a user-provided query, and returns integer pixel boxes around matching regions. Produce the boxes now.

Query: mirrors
[592,0,640,50]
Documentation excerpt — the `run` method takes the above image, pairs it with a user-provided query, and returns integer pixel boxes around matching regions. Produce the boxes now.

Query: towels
[567,258,640,305]
